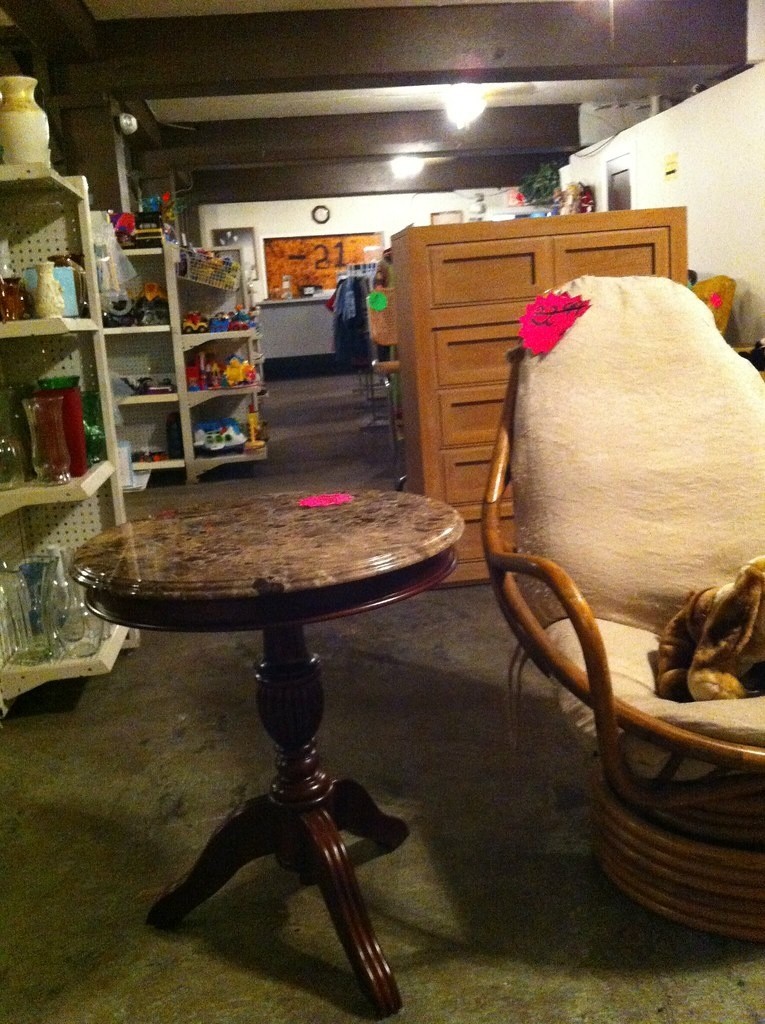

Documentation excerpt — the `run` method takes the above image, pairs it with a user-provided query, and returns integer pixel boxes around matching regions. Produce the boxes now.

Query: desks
[69,490,465,1019]
[255,291,335,358]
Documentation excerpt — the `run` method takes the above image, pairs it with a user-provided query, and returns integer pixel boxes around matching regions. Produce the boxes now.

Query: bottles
[281,276,292,301]
[0,376,105,492]
[0,255,64,318]
[0,543,116,667]
[0,75,51,163]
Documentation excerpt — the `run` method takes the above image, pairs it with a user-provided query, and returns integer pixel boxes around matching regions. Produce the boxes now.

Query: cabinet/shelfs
[393,206,689,585]
[0,161,267,717]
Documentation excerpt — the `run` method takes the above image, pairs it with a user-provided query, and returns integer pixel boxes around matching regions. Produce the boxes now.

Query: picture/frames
[211,227,259,282]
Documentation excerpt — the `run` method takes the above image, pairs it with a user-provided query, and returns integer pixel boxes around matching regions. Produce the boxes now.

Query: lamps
[114,114,137,134]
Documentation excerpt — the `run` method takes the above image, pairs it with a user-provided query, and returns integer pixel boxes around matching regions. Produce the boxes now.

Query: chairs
[479,275,765,943]
[365,288,401,432]
